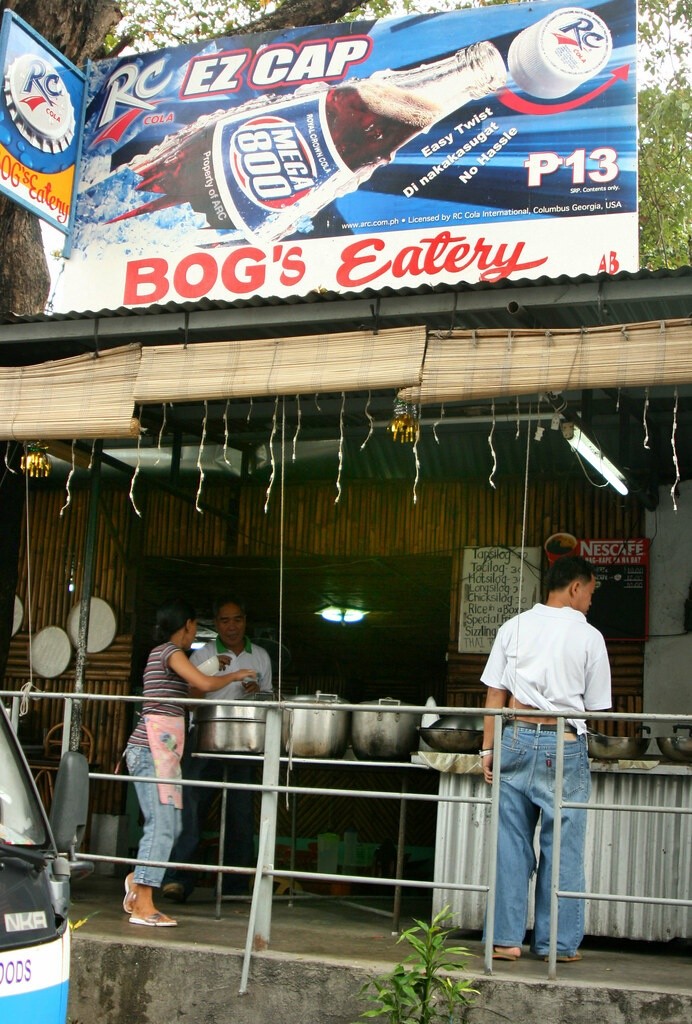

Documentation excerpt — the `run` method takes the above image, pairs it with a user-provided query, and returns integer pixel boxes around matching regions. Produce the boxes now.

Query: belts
[505,720,577,733]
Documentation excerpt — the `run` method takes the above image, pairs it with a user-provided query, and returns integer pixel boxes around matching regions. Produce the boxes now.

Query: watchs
[479,748,493,758]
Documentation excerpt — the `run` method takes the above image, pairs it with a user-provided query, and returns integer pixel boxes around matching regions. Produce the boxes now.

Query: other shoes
[544,950,583,962]
[492,948,522,961]
[161,883,188,903]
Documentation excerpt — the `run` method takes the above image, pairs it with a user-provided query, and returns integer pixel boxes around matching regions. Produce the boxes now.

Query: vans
[0,697,91,1024]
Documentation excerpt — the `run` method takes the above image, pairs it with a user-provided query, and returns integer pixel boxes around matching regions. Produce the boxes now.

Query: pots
[654,725,692,762]
[586,725,651,758]
[351,696,422,762]
[192,704,267,755]
[279,690,351,759]
[415,714,484,755]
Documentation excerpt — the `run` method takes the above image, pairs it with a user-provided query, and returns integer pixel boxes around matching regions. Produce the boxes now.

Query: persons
[121,598,260,926]
[480,554,614,960]
[160,600,274,901]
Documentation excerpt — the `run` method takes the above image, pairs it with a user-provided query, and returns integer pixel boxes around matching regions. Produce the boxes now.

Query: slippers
[123,876,136,913]
[129,913,177,926]
